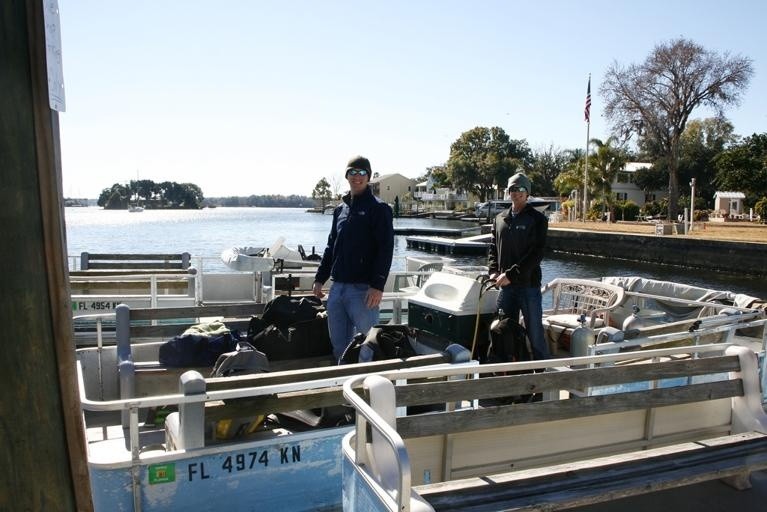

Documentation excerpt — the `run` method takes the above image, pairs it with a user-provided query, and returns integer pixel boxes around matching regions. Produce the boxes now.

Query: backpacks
[338,324,417,366]
[477,308,544,407]
[263,402,356,429]
[158,321,238,367]
[245,293,334,361]
[202,342,270,447]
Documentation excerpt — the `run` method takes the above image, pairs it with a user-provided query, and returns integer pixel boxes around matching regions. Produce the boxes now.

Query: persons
[488,173,547,402]
[312,159,394,362]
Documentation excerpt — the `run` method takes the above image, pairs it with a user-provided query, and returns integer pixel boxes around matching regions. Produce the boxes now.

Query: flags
[585,80,591,122]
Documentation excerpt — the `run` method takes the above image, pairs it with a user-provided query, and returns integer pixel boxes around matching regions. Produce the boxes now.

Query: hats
[508,173,531,195]
[345,156,371,181]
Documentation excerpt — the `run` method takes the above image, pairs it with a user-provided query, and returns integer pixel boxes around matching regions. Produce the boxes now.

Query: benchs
[80,251,196,297]
[519,278,625,354]
[166,344,471,450]
[114,304,338,429]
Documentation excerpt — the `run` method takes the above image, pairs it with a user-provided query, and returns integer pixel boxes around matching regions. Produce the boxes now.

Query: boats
[128,206,146,213]
[64,243,509,350]
[218,236,324,272]
[73,274,765,512]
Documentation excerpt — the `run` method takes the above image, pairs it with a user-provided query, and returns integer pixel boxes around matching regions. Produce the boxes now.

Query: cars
[474,188,557,218]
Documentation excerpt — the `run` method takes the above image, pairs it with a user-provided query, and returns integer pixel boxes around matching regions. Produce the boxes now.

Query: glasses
[349,169,367,176]
[510,186,526,192]
